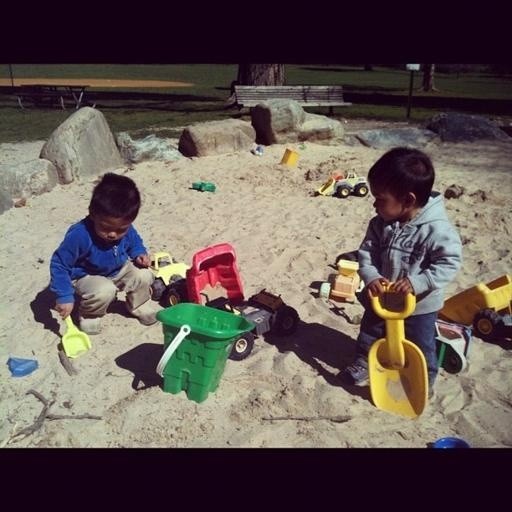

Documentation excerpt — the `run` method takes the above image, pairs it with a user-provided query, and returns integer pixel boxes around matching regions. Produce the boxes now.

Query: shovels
[62,314,92,359]
[368,281,429,420]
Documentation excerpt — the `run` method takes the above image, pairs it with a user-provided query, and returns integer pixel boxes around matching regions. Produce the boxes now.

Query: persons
[332,149,463,401]
[47,171,160,335]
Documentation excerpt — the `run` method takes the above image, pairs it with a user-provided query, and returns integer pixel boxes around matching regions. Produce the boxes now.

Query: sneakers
[81,316,103,334]
[338,363,368,385]
[125,300,162,325]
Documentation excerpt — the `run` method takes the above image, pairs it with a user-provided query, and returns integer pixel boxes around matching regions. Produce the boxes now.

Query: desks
[21,84,90,108]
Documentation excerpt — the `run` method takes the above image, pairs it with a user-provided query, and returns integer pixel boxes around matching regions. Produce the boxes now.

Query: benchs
[14,92,98,110]
[233,85,353,117]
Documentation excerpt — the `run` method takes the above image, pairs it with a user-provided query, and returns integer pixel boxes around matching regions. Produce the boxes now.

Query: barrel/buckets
[152,301,255,402]
